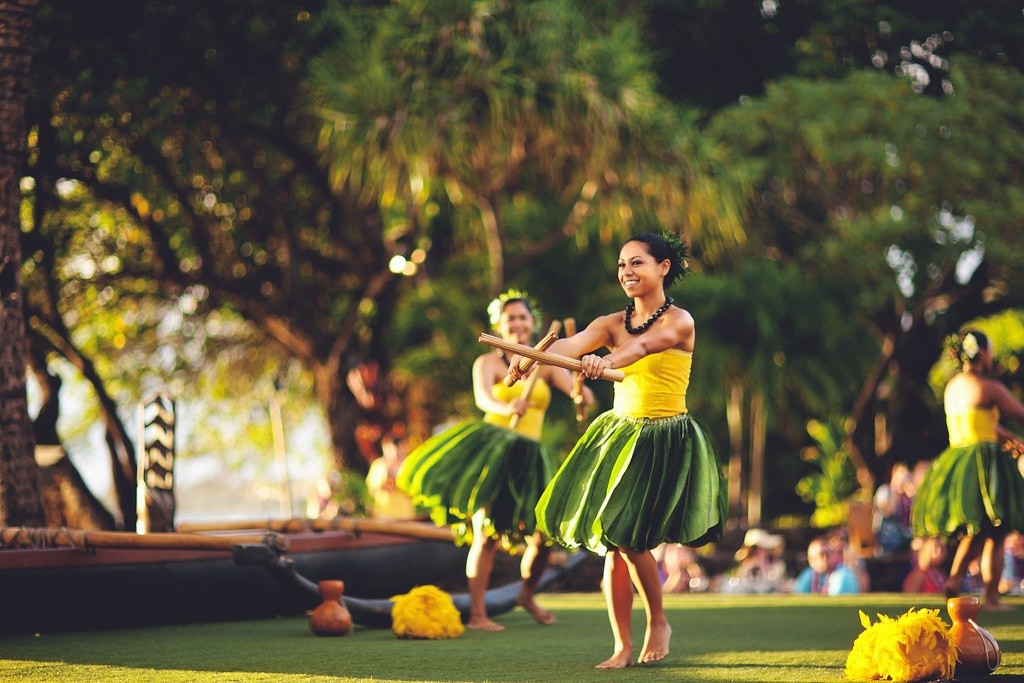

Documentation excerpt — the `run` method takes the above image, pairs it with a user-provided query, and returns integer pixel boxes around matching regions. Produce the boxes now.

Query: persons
[392,290,560,631]
[359,419,1024,597]
[509,233,722,672]
[912,330,1024,607]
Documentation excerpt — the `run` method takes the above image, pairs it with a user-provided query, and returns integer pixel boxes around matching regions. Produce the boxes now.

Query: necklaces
[625,298,675,335]
[497,346,509,365]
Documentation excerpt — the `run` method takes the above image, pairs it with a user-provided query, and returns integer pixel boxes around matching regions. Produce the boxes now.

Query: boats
[0,516,550,637]
